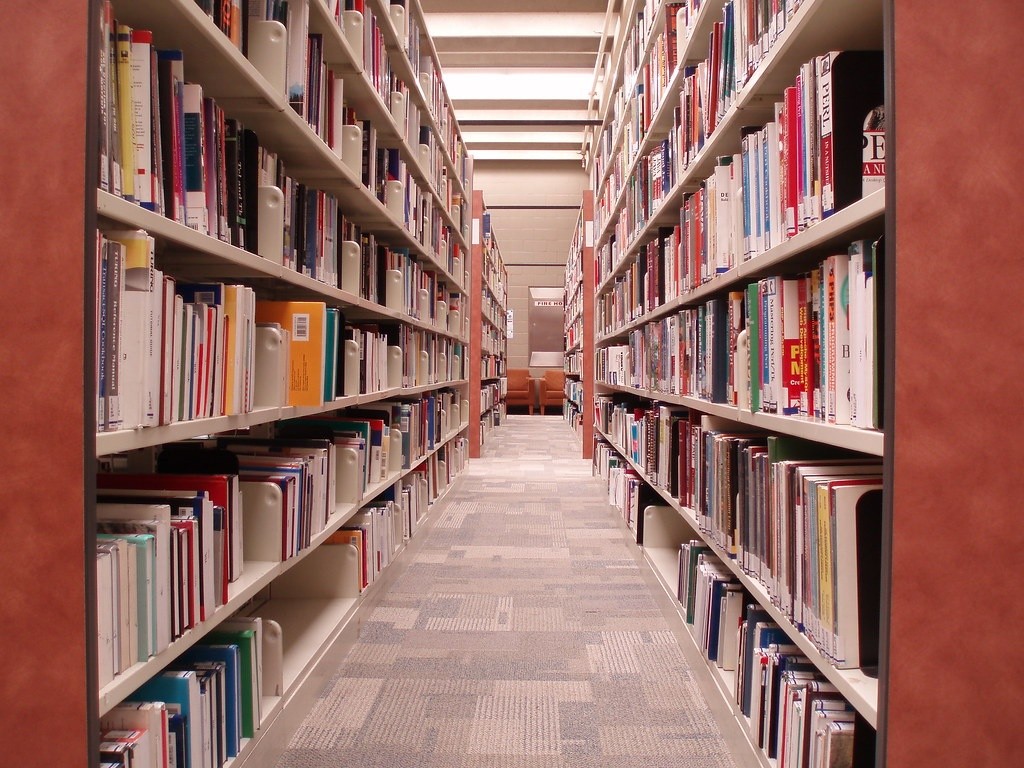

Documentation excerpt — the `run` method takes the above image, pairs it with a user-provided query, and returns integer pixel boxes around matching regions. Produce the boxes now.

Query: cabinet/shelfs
[563,0,1024,768]
[0,0,507,768]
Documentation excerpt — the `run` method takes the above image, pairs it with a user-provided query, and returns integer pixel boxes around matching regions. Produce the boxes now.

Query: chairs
[507,369,536,415]
[538,370,565,415]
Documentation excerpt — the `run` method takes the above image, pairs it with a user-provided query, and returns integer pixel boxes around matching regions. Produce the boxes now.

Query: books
[90,0,511,768]
[560,0,884,768]
